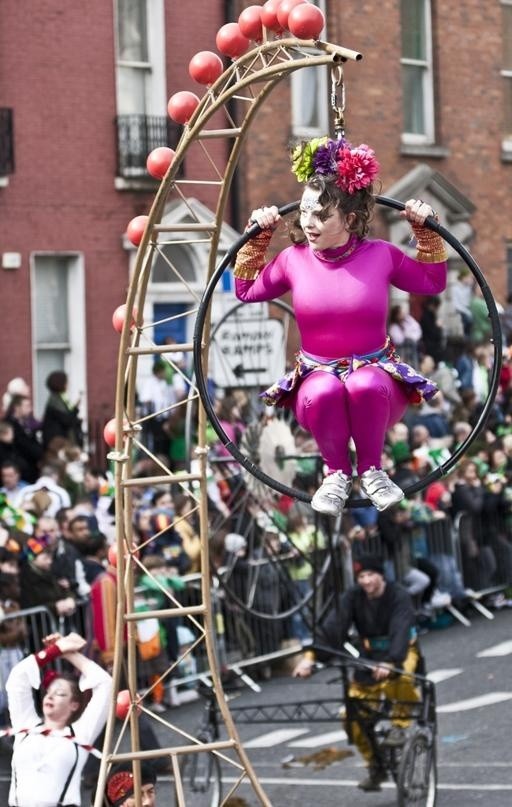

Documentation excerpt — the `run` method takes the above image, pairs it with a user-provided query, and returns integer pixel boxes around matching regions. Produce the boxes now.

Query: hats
[353,554,384,580]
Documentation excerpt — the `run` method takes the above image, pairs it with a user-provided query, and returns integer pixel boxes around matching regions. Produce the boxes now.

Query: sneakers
[357,467,403,512]
[358,770,389,791]
[310,472,353,518]
[383,726,408,749]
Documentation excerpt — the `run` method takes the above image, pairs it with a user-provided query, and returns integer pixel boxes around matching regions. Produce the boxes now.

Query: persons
[233,137,449,516]
[290,552,435,792]
[5,630,114,807]
[0,268,512,713]
